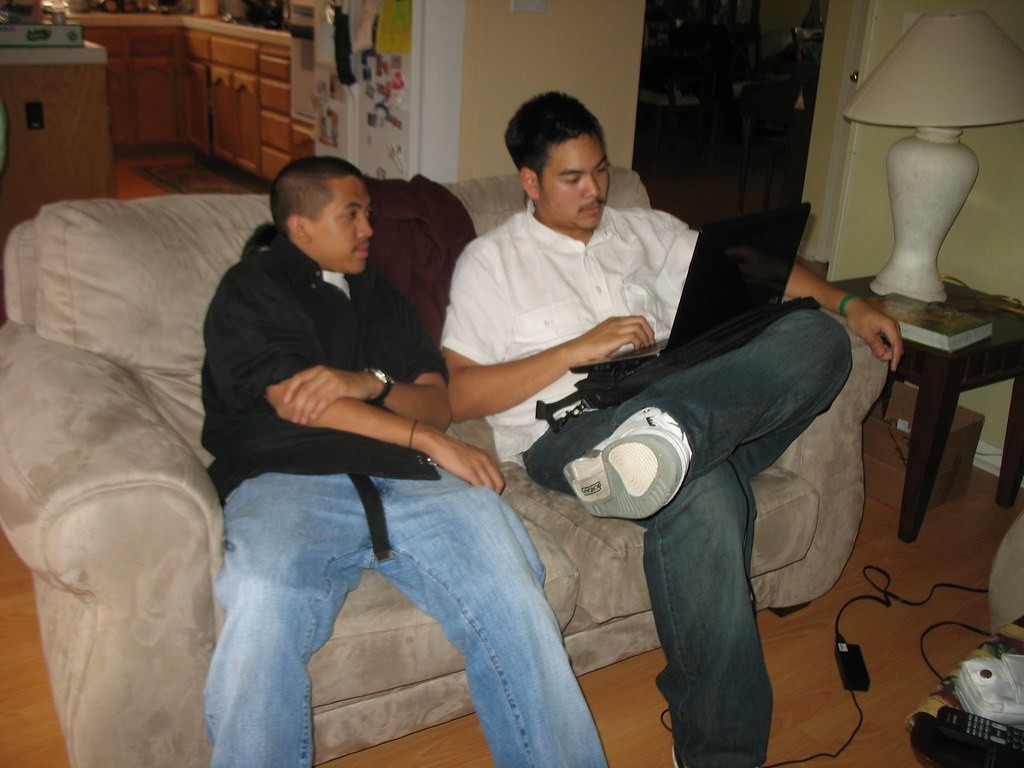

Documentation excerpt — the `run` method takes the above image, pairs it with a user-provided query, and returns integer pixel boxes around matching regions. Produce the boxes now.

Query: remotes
[937,706,1024,757]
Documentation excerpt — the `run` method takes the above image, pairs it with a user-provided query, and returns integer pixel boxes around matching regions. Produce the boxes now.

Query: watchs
[363,366,396,408]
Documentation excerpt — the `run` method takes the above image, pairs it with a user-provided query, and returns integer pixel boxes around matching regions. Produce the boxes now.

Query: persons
[202,156,610,768]
[442,89,906,768]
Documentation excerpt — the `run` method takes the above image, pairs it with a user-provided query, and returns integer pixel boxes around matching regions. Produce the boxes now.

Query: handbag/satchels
[535,294,821,432]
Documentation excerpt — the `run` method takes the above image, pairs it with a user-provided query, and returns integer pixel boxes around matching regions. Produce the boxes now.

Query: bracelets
[839,292,865,318]
[408,420,418,450]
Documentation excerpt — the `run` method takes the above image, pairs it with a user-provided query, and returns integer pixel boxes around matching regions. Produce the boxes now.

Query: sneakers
[563,406,693,519]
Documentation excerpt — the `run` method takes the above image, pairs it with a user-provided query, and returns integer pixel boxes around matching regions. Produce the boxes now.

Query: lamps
[841,9,1024,304]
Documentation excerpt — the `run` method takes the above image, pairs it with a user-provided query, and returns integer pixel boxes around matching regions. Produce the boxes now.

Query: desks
[831,276,1024,542]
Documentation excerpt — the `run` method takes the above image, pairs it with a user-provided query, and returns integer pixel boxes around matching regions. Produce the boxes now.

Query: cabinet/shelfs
[82,26,316,185]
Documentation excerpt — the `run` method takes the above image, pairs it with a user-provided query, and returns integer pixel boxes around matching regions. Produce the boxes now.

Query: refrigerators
[314,0,465,187]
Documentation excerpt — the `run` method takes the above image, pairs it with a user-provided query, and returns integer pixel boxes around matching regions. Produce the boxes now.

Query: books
[869,293,993,353]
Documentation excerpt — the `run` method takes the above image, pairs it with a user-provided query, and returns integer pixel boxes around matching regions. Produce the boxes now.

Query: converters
[835,643,871,693]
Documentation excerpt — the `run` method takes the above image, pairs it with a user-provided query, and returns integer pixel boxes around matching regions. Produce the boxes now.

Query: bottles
[52,0,64,24]
[319,71,338,147]
[796,0,823,66]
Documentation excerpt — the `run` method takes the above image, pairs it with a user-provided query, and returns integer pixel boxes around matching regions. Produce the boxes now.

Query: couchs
[0,166,890,768]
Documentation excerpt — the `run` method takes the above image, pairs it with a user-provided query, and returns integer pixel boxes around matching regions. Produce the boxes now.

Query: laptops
[569,203,813,375]
